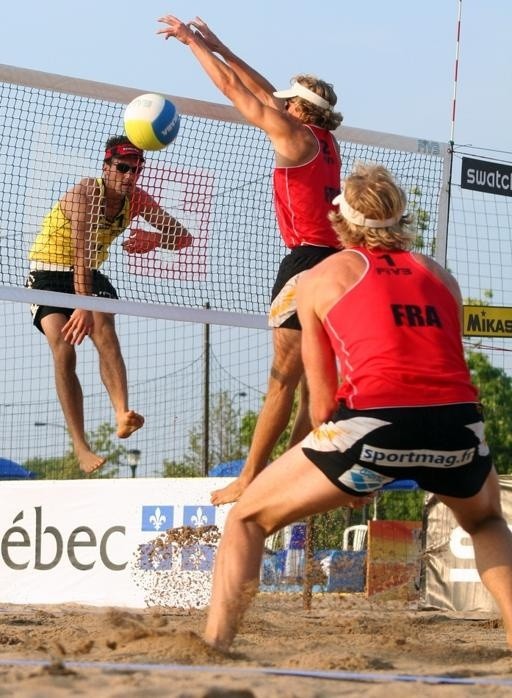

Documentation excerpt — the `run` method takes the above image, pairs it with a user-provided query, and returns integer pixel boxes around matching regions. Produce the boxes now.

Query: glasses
[112,163,143,174]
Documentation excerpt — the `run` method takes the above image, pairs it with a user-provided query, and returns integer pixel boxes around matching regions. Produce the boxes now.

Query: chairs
[321,524,368,586]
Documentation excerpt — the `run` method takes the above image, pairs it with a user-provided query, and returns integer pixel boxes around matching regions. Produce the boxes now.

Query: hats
[273,81,334,112]
[104,144,145,162]
[332,190,402,228]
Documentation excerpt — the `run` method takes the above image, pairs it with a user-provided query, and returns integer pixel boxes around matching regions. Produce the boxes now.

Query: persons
[197,159,512,661]
[156,14,342,509]
[24,134,194,475]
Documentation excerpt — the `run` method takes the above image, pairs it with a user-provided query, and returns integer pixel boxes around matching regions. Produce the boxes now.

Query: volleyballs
[122,94,181,152]
